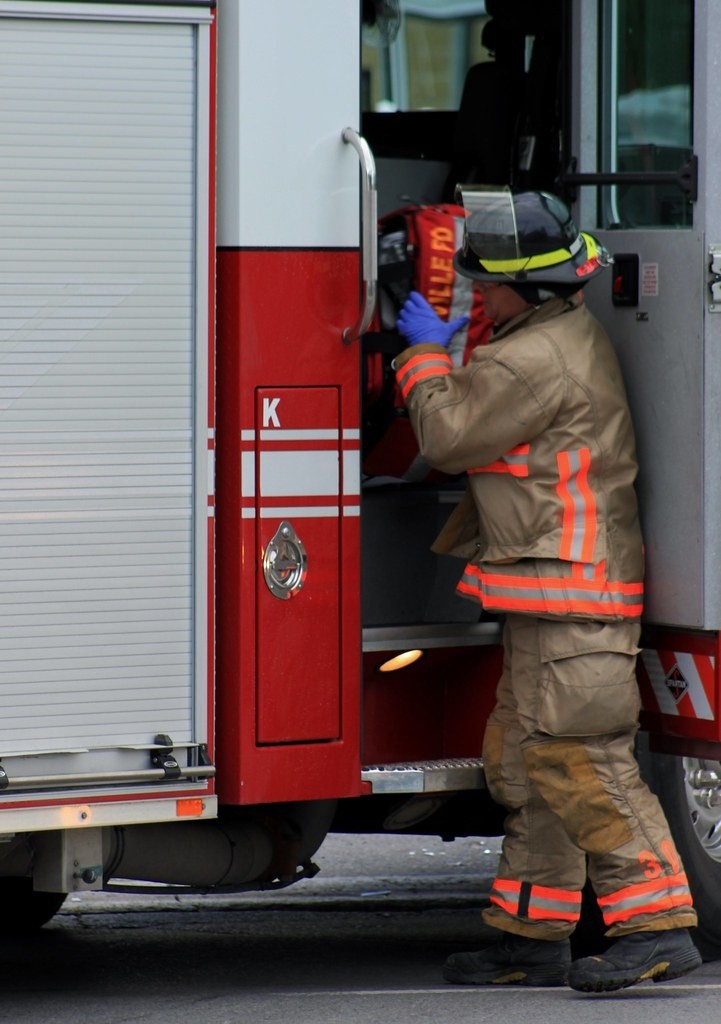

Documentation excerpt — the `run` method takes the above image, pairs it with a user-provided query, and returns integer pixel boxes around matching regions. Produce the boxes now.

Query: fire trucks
[0,0,721,948]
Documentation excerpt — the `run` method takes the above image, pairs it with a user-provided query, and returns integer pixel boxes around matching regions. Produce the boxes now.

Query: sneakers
[442,927,570,986]
[568,925,703,992]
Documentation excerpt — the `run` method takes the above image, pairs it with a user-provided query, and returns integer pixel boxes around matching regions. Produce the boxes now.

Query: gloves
[397,290,471,350]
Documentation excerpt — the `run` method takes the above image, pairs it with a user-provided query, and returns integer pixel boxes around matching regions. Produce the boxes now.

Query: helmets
[453,183,613,285]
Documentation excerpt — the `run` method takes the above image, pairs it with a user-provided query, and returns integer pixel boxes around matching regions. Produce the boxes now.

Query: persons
[391,189,705,994]
